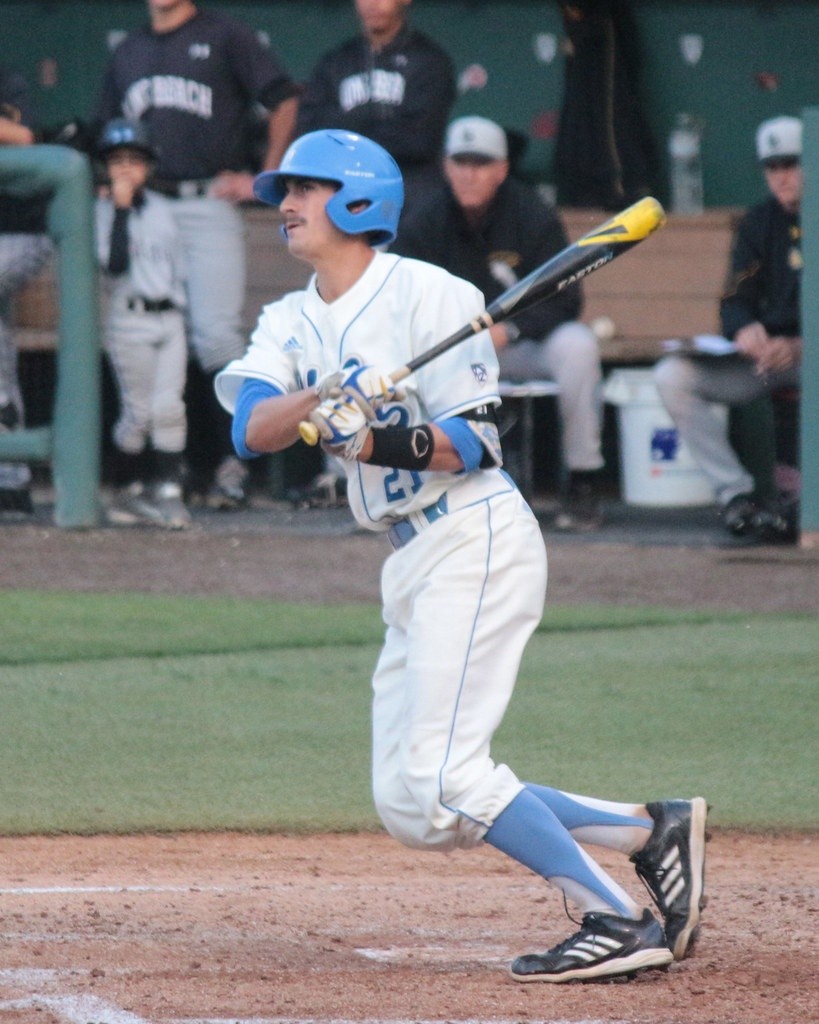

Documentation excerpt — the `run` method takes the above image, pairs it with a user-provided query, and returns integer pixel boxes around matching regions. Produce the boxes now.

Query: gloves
[312,366,421,463]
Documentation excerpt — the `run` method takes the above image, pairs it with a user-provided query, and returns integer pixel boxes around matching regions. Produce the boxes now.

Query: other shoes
[723,489,798,543]
[189,488,290,514]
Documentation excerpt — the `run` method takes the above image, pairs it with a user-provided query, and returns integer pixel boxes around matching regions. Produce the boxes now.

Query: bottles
[667,112,704,216]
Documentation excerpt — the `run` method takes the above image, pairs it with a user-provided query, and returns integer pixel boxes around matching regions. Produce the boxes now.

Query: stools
[500,379,558,514]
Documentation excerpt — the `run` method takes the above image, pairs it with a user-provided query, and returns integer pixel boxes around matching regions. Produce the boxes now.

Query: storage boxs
[605,368,728,505]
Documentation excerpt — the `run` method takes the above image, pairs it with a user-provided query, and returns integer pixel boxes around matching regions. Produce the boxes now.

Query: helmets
[252,129,403,249]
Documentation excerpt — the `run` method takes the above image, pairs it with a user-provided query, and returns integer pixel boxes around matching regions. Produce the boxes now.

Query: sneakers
[629,796,713,961]
[288,476,348,509]
[510,909,674,984]
[556,499,608,531]
[98,482,193,533]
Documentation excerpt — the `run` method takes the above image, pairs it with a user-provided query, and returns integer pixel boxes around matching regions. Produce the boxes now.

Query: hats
[444,115,508,162]
[99,117,163,162]
[755,114,801,158]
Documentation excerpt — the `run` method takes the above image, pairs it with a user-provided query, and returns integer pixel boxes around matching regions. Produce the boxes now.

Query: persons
[211,133,710,979]
[390,116,606,533]
[90,120,191,529]
[651,117,803,542]
[298,0,456,505]
[94,2,297,512]
[1,59,90,520]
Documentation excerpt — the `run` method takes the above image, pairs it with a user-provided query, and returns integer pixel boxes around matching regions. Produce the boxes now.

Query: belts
[124,295,178,314]
[149,177,216,199]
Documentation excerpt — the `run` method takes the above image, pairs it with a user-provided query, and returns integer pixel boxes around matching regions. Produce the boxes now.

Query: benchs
[12,204,733,363]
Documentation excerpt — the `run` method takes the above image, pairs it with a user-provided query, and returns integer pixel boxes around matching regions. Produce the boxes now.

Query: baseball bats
[297,195,668,445]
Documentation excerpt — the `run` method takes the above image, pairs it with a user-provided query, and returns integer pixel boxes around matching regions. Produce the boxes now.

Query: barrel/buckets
[608,367,729,507]
